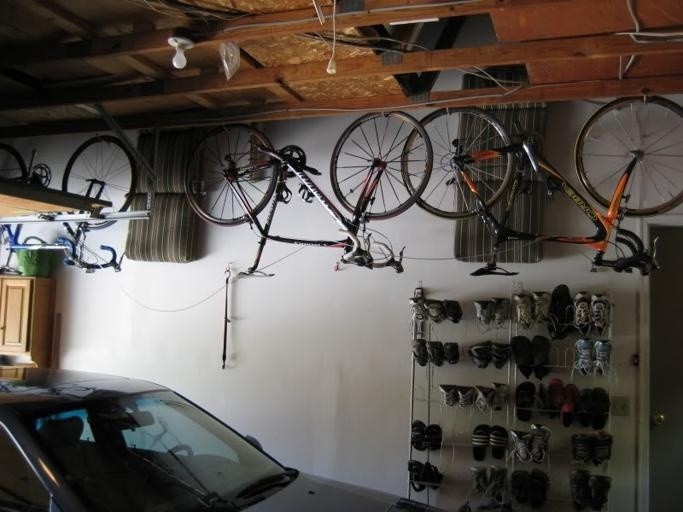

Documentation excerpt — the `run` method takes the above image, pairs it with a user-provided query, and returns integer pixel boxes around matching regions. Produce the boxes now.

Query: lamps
[167,25,195,69]
[326,0,338,75]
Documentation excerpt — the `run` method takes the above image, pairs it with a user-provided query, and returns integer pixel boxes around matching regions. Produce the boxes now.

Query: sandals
[408,460,425,492]
[423,461,443,489]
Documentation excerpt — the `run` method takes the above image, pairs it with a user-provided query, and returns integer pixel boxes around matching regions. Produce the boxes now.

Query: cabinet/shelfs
[407,297,616,512]
[0,274,55,354]
[0,364,48,383]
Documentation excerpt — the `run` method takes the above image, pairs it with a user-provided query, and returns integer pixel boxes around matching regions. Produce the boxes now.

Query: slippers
[471,424,490,461]
[489,425,508,459]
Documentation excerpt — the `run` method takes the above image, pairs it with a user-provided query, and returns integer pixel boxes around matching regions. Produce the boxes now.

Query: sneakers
[590,475,612,512]
[532,336,552,381]
[475,385,495,411]
[491,342,512,369]
[409,298,425,321]
[570,469,591,512]
[572,291,593,337]
[575,338,594,376]
[509,336,534,379]
[560,384,579,425]
[424,300,446,323]
[572,433,591,464]
[474,300,494,325]
[546,378,563,418]
[444,300,462,324]
[468,340,491,369]
[507,430,533,464]
[485,464,507,497]
[510,470,530,503]
[470,466,488,496]
[457,386,475,407]
[411,420,426,451]
[592,431,613,467]
[513,294,538,329]
[492,298,510,327]
[532,291,550,323]
[491,383,508,410]
[529,468,548,510]
[528,424,551,465]
[424,424,442,450]
[411,339,428,366]
[426,341,444,367]
[590,294,610,337]
[439,384,459,406]
[515,381,535,422]
[591,387,609,430]
[592,339,611,376]
[443,343,459,364]
[579,388,591,427]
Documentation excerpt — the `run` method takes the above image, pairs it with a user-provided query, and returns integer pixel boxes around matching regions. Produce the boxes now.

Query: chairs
[30,417,84,472]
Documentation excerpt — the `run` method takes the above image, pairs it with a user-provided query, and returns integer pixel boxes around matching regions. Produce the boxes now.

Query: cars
[0,373,441,511]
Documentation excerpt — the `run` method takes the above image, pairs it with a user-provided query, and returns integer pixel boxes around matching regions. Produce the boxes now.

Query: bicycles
[181,110,433,277]
[404,97,682,277]
[0,132,136,274]
